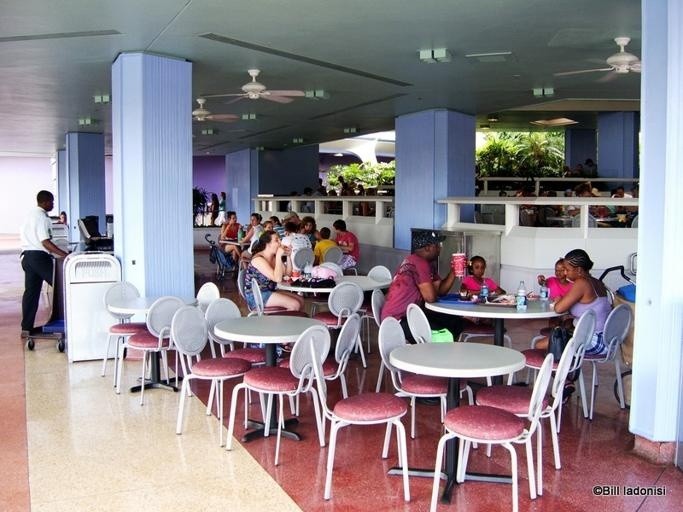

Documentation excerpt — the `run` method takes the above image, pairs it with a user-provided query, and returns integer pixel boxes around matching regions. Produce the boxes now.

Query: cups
[451,253,465,277]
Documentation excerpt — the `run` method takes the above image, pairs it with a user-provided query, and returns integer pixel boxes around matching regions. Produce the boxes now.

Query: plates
[483,301,517,306]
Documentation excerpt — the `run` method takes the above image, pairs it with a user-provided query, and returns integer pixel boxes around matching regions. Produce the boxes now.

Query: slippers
[279,344,292,352]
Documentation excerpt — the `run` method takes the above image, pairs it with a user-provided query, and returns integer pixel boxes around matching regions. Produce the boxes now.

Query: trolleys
[599,265,636,411]
[23,255,66,353]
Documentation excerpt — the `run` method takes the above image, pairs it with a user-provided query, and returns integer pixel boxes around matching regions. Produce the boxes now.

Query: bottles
[289,268,302,281]
[538,281,549,303]
[106,216,113,239]
[515,279,527,310]
[237,227,243,242]
[479,279,487,298]
[303,261,312,279]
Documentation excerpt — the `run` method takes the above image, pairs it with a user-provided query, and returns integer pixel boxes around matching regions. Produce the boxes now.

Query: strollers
[204,233,240,282]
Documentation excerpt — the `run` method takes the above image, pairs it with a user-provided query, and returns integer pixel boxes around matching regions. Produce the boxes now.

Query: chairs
[225,324,334,467]
[459,334,578,499]
[519,307,599,436]
[307,335,412,503]
[375,315,479,467]
[171,306,250,448]
[99,236,572,431]
[426,352,554,512]
[579,304,632,421]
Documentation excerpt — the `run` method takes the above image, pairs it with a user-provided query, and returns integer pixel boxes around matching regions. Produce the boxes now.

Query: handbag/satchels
[292,275,335,288]
[549,326,566,361]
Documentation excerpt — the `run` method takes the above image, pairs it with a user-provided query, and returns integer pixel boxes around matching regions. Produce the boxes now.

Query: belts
[20,250,43,258]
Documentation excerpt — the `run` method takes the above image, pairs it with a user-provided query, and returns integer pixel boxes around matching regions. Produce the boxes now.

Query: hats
[412,232,447,250]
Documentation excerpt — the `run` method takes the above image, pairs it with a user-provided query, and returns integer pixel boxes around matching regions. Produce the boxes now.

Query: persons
[378,231,468,407]
[56,210,66,223]
[208,155,638,229]
[534,248,615,407]
[19,189,67,340]
[536,257,575,334]
[218,210,360,354]
[461,254,506,329]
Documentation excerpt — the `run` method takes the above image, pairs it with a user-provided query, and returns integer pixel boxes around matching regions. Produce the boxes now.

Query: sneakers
[563,382,575,405]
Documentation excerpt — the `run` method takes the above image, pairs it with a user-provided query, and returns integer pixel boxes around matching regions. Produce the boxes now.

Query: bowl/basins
[526,295,538,301]
[459,296,470,301]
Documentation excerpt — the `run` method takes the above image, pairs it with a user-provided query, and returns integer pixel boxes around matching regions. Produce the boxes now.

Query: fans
[552,35,642,80]
[199,67,306,105]
[191,94,241,126]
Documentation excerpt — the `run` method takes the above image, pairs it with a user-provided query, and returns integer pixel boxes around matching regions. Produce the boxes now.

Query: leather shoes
[415,397,439,406]
